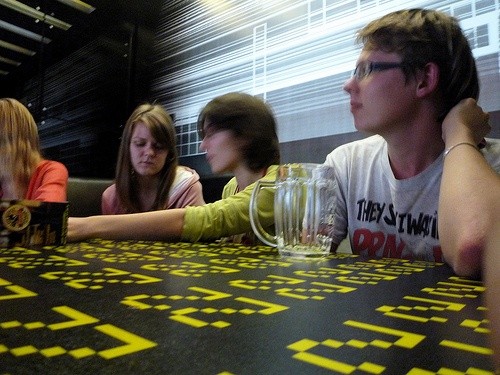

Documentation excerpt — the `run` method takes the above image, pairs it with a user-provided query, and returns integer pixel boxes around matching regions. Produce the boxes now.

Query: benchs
[66,176,233,218]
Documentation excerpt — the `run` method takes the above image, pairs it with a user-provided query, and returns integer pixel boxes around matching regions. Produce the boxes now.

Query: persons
[66,92,309,242]
[102,104,205,216]
[0,98,67,201]
[303,9,499,276]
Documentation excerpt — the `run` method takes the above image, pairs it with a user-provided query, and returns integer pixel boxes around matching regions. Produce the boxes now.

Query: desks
[0,240,498,375]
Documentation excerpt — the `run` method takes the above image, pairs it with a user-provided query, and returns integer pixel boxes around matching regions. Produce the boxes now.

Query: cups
[16,200,50,249]
[42,202,71,246]
[249,162,337,258]
[0,199,17,248]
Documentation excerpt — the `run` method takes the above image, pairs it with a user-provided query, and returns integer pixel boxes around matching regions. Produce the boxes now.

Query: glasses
[353,59,406,80]
[199,123,220,138]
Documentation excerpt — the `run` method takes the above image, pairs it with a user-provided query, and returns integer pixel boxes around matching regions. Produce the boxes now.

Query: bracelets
[444,141,480,156]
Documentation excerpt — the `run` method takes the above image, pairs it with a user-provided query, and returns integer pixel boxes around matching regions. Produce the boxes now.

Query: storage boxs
[0,200,70,248]
